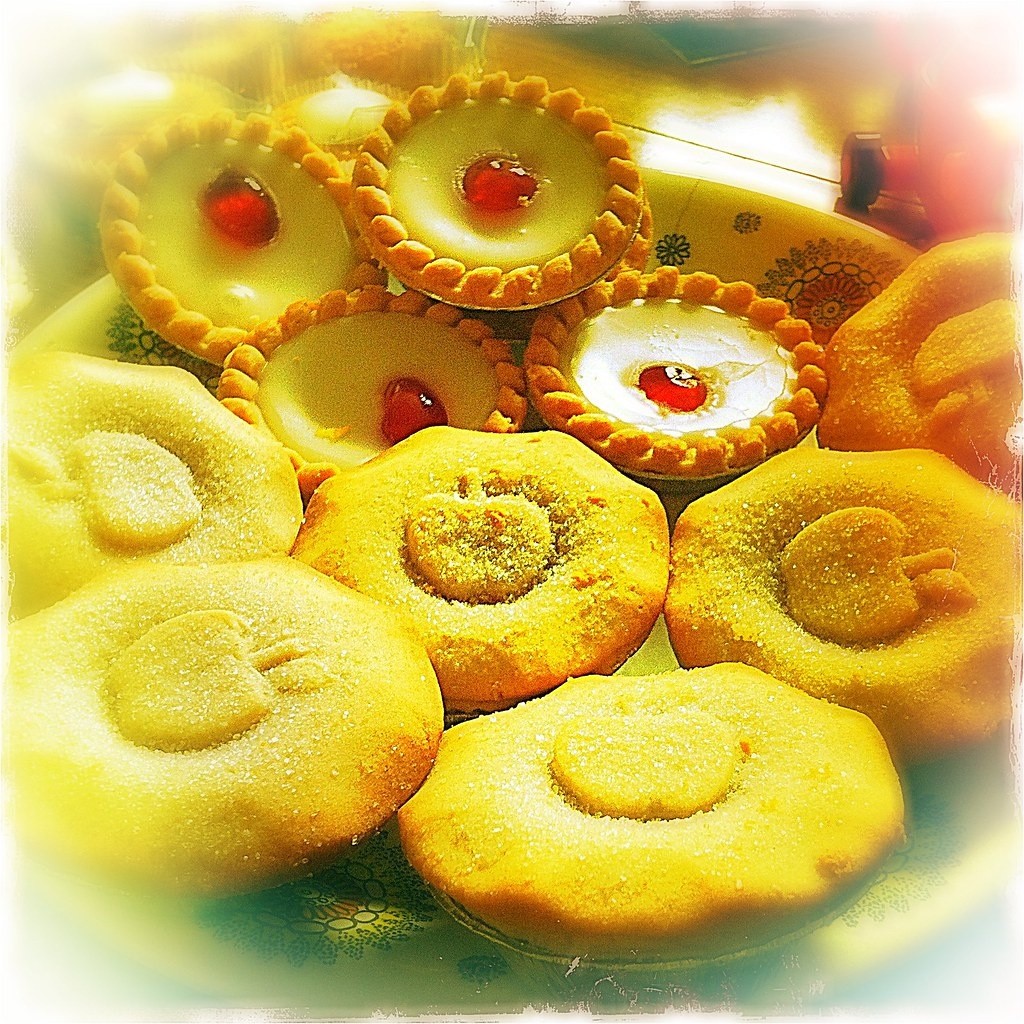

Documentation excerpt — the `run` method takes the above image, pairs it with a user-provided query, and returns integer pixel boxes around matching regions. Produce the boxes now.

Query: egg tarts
[87,71,828,492]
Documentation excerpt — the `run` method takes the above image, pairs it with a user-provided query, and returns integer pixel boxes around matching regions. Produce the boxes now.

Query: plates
[0,166,1024,1022]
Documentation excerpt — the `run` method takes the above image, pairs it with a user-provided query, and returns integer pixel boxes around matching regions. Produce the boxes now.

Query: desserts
[2,232,1024,976]
